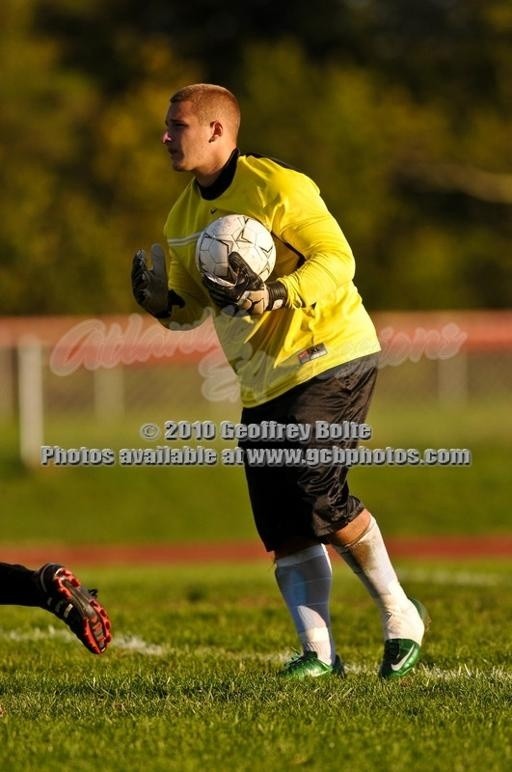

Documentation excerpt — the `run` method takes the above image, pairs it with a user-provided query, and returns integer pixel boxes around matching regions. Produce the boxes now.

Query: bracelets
[266,278,288,317]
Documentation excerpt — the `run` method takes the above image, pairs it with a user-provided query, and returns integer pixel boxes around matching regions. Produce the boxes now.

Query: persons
[133,80,427,692]
[2,557,114,658]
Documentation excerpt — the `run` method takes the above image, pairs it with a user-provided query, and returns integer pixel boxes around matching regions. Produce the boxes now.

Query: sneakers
[32,561,112,652]
[377,597,431,683]
[275,652,345,684]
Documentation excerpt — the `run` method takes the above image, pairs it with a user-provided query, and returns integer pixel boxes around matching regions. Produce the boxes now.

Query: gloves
[200,250,288,318]
[132,244,185,320]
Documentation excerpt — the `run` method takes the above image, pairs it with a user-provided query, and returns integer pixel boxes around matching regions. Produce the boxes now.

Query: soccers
[194,212,276,287]
